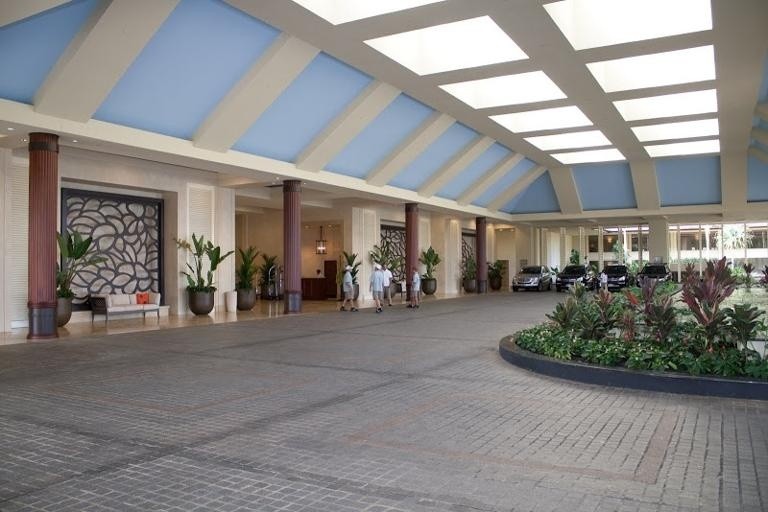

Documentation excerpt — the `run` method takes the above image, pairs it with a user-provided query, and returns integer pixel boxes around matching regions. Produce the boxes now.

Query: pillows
[111,294,130,304]
[136,292,149,304]
[149,292,160,305]
[129,294,137,304]
[91,293,111,306]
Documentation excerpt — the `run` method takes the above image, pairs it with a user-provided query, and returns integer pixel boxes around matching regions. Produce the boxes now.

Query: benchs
[90,291,161,328]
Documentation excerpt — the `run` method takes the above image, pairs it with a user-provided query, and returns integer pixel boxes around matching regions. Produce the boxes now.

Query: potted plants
[461,255,476,293]
[418,245,441,295]
[260,252,277,300]
[172,232,235,316]
[487,259,504,290]
[236,245,260,311]
[340,250,361,301]
[56,230,108,327]
[370,240,403,298]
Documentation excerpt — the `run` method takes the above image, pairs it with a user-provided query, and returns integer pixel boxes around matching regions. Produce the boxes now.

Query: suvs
[635,262,673,288]
[554,264,602,293]
[599,264,631,292]
[511,265,553,292]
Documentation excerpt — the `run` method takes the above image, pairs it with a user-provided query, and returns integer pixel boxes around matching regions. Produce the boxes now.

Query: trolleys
[267,265,283,301]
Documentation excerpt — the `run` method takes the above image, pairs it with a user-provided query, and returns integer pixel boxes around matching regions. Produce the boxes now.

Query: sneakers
[407,304,412,308]
[340,306,347,311]
[376,308,382,312]
[413,305,419,308]
[351,307,358,312]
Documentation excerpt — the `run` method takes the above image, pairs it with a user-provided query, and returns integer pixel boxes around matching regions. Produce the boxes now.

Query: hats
[375,262,382,269]
[345,265,353,271]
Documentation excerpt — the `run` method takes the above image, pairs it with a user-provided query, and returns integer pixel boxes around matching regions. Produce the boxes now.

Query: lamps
[316,226,328,254]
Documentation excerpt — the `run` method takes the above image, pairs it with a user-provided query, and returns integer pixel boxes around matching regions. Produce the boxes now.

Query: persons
[406,266,422,308]
[316,269,326,278]
[369,264,384,313]
[381,264,394,306]
[597,269,608,289]
[340,265,359,312]
